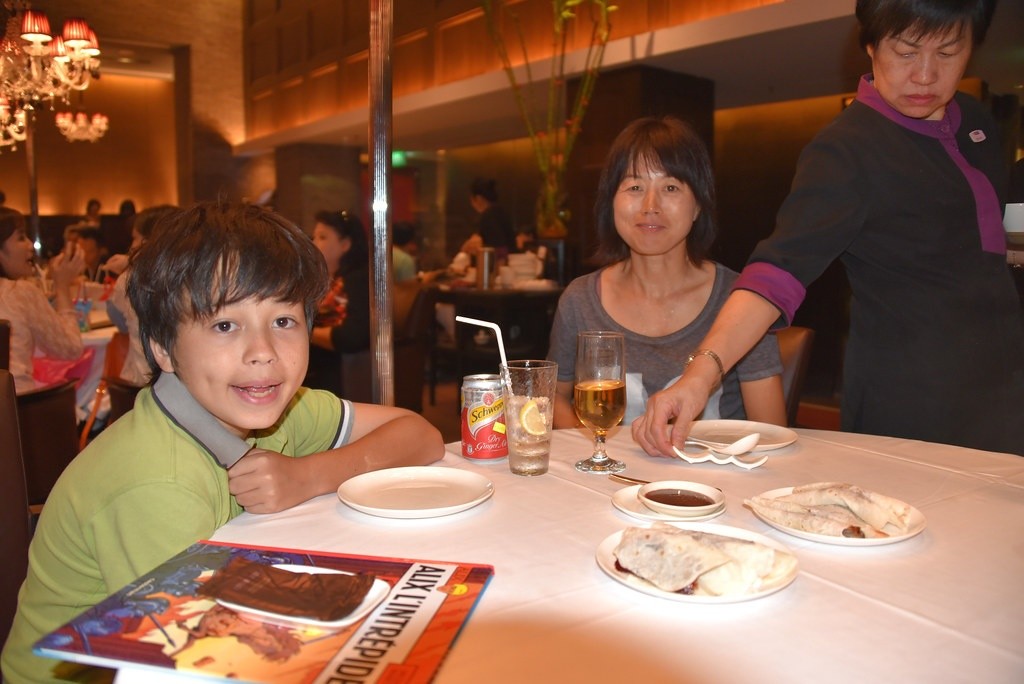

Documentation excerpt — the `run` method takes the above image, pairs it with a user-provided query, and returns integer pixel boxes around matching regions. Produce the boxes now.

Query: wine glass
[572,329,627,474]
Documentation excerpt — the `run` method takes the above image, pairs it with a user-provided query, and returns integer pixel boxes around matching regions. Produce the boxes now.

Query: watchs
[686,349,726,384]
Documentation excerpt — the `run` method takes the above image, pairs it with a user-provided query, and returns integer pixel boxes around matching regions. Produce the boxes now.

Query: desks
[75,324,112,425]
[425,284,566,415]
[111,425,1024,684]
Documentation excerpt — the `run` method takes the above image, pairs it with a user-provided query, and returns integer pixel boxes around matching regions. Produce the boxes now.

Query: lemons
[519,400,548,435]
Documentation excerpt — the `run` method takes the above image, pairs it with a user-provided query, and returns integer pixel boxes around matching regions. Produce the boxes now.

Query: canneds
[460,374,511,463]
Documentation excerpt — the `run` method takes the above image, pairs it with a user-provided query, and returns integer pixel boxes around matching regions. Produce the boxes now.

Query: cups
[501,360,559,477]
[477,248,495,292]
[498,266,514,290]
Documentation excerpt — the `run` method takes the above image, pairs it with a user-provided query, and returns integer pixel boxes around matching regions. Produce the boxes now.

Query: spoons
[684,434,760,454]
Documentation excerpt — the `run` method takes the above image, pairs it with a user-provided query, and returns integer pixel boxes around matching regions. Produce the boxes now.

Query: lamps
[0,0,111,151]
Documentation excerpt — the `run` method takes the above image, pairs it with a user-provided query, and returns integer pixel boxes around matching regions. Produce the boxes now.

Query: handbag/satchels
[49,282,92,333]
[99,331,129,384]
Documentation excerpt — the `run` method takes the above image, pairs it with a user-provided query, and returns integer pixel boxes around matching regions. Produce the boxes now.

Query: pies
[613,520,797,598]
[741,481,913,540]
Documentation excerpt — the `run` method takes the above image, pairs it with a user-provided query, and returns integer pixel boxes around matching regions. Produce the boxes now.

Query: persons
[0,203,446,684]
[531,119,786,429]
[620,0,1023,458]
[0,176,538,439]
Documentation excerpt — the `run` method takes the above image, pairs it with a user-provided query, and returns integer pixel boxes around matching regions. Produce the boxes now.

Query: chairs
[332,281,429,412]
[0,317,149,649]
[774,325,819,429]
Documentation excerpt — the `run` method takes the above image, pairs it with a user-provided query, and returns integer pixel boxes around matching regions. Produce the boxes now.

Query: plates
[611,484,726,522]
[687,420,798,451]
[338,466,494,519]
[640,480,724,516]
[597,521,799,603]
[753,486,927,546]
[214,564,391,633]
